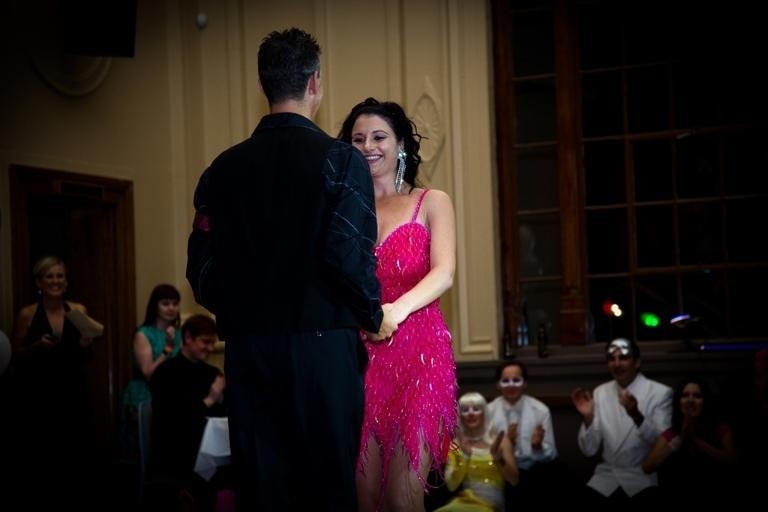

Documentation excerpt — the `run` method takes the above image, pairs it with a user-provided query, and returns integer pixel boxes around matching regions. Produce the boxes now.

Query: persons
[482,361,560,512]
[642,380,737,512]
[11,255,97,512]
[570,337,676,511]
[122,284,184,472]
[334,97,459,512]
[432,392,520,512]
[185,28,389,511]
[148,314,235,491]
